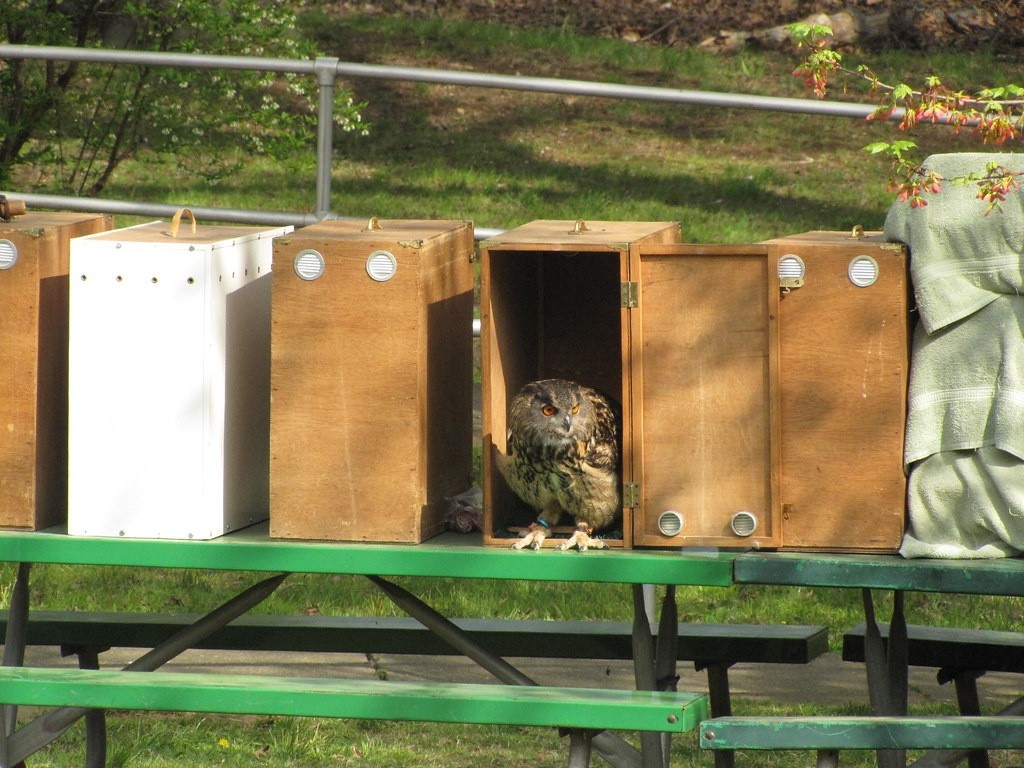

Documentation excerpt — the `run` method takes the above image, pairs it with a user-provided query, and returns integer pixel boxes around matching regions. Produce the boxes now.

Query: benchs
[0,609,1024,768]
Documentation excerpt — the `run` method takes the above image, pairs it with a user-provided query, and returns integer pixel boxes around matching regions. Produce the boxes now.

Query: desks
[2,522,743,768]
[733,554,1024,768]
